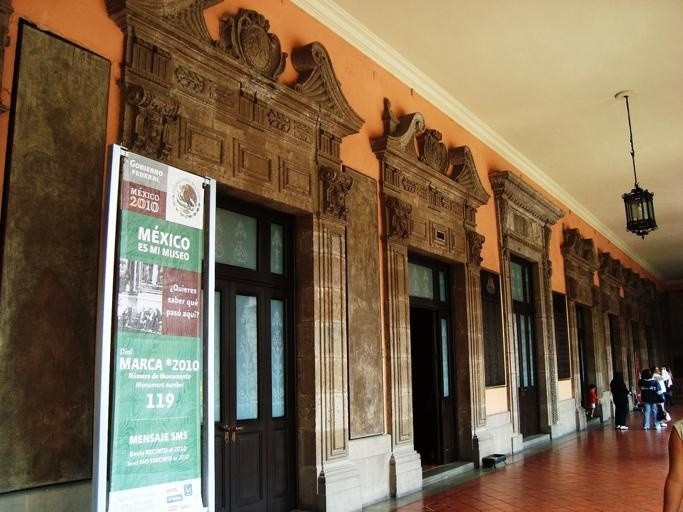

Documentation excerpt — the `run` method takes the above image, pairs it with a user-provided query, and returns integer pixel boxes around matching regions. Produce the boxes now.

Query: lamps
[614,90,659,241]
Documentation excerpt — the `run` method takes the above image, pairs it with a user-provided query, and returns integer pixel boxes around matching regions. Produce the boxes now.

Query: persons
[585,384,599,418]
[609,371,629,429]
[637,363,673,431]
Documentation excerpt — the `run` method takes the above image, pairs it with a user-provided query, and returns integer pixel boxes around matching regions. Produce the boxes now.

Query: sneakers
[617,425,628,429]
[643,417,671,430]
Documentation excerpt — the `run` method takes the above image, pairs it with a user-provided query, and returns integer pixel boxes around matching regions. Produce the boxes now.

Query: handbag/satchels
[657,405,666,420]
